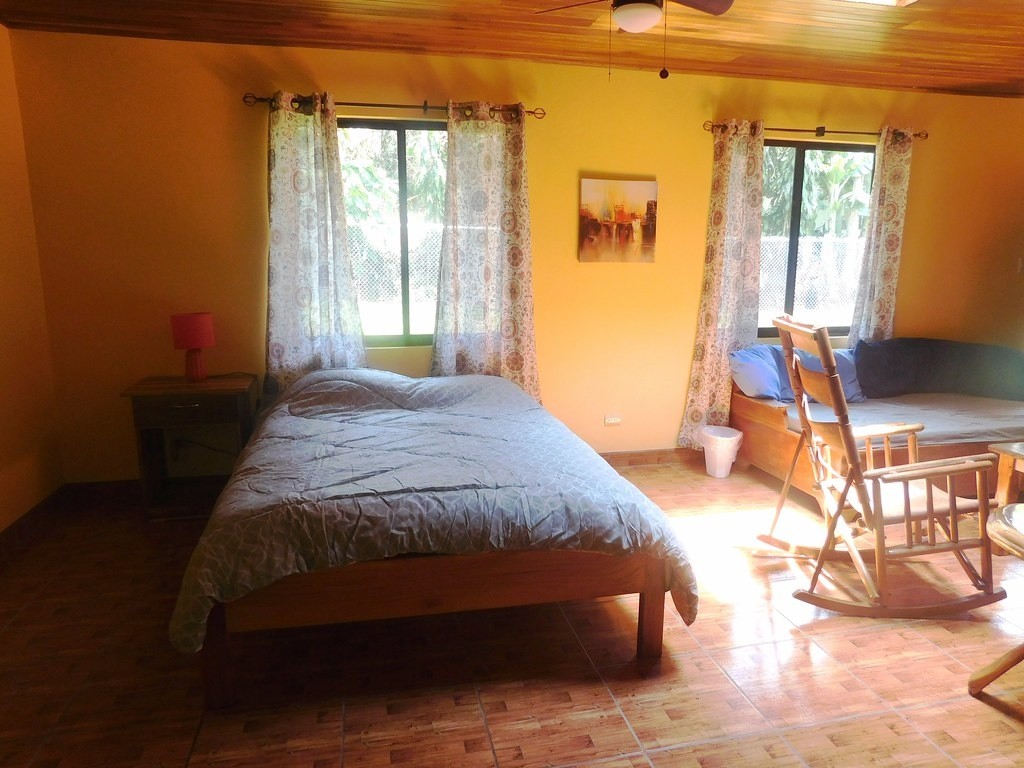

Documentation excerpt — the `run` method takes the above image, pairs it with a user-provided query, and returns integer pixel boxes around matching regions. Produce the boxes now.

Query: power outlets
[605,415,623,425]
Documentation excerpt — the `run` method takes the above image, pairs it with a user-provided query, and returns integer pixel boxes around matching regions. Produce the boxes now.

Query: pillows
[730,343,866,404]
[853,339,1024,402]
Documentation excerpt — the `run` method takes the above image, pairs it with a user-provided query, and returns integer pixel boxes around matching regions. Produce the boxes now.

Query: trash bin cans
[701,425,743,478]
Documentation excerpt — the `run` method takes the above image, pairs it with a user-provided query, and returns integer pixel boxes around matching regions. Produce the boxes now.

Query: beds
[729,337,1024,522]
[166,368,699,712]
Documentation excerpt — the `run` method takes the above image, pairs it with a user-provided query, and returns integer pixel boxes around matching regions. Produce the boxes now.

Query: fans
[534,0,735,84]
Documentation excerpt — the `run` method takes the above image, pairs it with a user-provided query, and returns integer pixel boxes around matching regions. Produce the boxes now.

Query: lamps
[171,311,217,378]
[610,0,663,33]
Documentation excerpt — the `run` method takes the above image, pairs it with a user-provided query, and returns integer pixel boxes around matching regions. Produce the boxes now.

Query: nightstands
[121,375,261,507]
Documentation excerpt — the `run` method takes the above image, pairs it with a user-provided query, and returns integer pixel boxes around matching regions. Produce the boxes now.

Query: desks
[988,442,1024,557]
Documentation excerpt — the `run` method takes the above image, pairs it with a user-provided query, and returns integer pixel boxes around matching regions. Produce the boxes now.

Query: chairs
[967,504,1024,725]
[758,312,1010,619]
